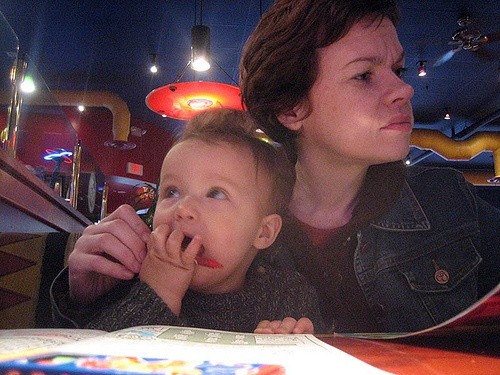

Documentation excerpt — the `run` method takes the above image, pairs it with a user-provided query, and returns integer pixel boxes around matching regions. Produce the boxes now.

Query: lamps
[444,108,451,119]
[147,54,160,76]
[416,59,428,76]
[144,0,247,121]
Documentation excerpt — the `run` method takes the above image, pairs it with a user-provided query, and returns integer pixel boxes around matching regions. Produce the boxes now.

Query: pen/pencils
[191,256,224,269]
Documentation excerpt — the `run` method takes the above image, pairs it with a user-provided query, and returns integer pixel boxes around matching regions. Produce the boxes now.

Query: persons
[84,106,327,335]
[48,0,499,336]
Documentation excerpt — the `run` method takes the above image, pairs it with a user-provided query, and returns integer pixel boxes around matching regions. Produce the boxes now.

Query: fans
[433,6,500,67]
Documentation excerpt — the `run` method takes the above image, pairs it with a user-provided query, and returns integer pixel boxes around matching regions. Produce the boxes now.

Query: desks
[316,332,500,375]
